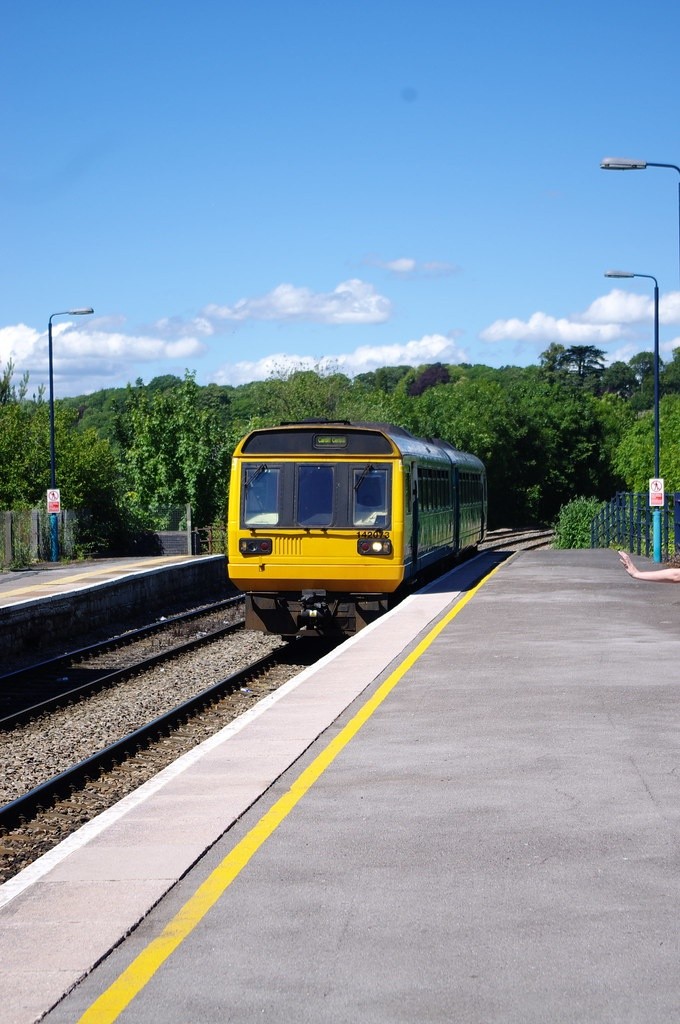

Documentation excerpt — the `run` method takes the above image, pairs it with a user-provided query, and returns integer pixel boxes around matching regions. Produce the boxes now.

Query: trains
[228,418,488,635]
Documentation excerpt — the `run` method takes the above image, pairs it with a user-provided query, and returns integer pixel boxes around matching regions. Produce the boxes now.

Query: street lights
[604,268,663,562]
[44,306,95,563]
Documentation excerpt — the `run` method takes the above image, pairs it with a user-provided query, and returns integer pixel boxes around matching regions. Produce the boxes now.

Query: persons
[617,550,680,583]
